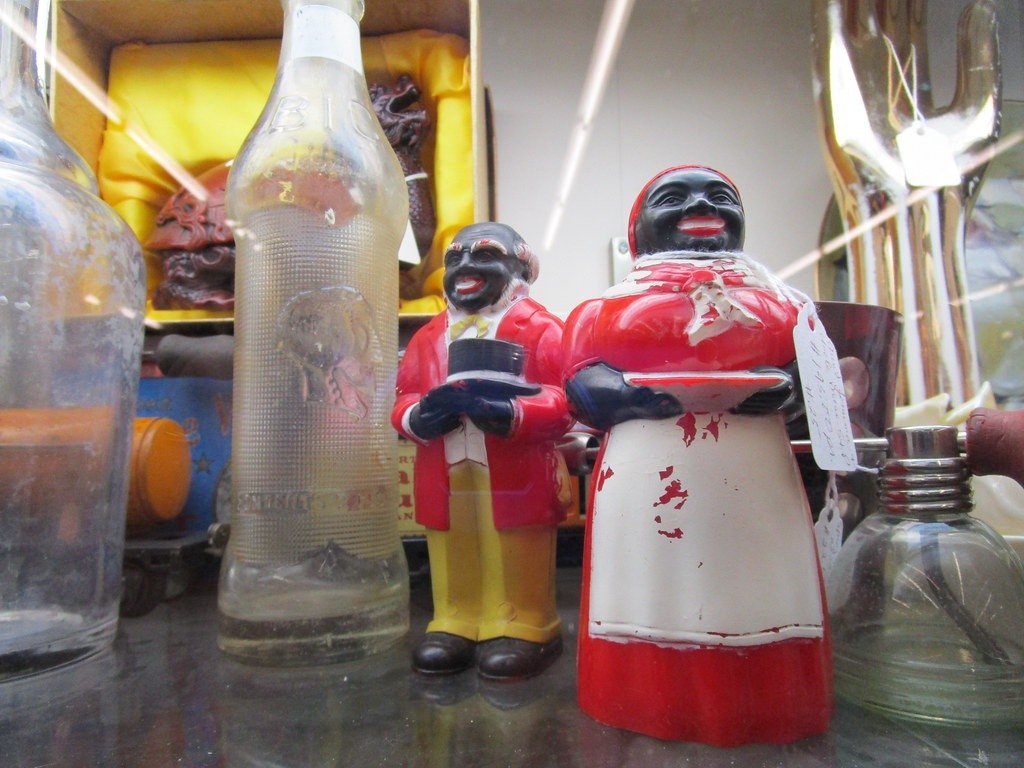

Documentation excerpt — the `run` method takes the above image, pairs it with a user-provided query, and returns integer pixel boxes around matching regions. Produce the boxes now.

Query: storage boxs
[13,374,238,537]
[43,2,493,336]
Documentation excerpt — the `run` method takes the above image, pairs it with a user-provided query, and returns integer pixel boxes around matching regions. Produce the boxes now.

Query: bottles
[0,1,147,683]
[813,425,1024,732]
[218,1,412,659]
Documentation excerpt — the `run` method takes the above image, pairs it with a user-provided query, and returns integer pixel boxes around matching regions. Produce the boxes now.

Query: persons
[390,220,579,682]
[559,163,831,745]
